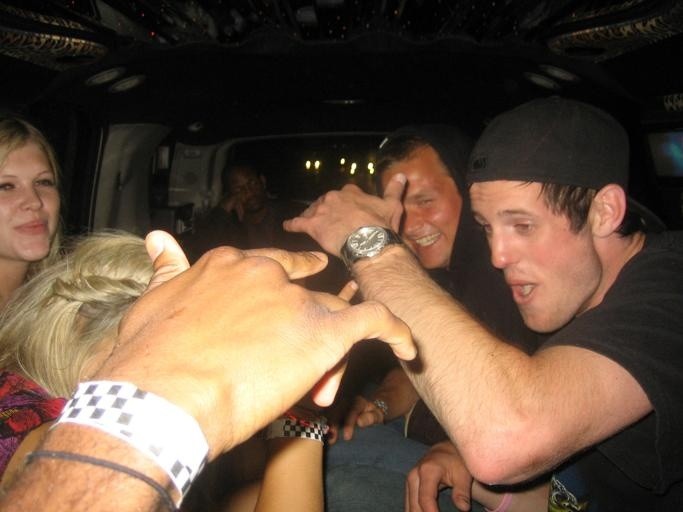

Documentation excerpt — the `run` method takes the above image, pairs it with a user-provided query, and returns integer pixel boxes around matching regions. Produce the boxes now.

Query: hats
[464,96,668,233]
